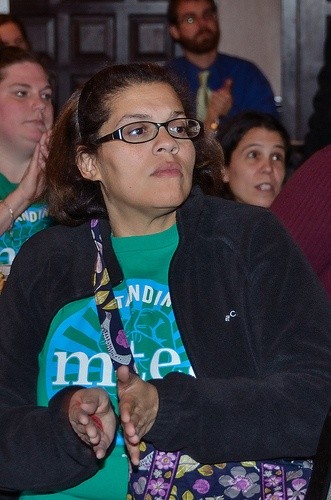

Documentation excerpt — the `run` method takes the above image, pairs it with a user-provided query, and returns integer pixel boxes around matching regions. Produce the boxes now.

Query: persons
[162,0,331,298]
[0,64,331,500]
[0,14,56,294]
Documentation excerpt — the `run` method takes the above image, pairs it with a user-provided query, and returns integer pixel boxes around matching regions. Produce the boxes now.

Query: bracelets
[2,200,14,229]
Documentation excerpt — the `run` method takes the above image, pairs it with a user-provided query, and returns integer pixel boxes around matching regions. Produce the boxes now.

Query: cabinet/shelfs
[9,0,183,121]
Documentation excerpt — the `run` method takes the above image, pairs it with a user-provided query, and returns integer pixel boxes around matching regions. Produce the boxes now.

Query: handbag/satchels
[117,449,313,500]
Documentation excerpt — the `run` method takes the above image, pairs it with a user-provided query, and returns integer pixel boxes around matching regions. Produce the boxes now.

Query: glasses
[176,11,217,24]
[96,118,204,144]
